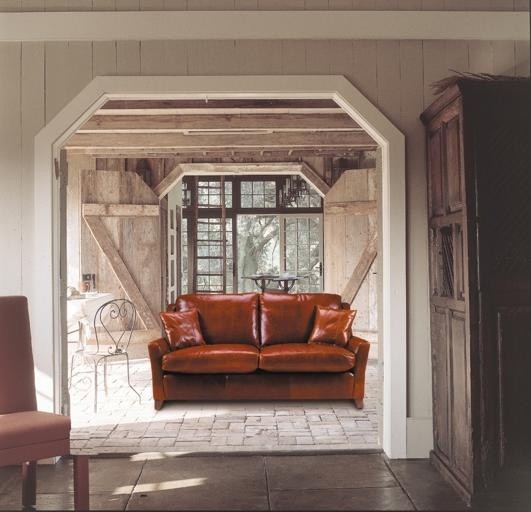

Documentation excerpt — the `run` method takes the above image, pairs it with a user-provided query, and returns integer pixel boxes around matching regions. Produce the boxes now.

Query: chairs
[69,297,143,413]
[1,294,93,512]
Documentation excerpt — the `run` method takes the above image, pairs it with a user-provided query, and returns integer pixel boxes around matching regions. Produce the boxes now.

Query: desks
[66,289,112,383]
[240,273,308,295]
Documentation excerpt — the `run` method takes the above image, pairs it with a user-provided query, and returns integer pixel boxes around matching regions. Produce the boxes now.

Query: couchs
[144,291,373,411]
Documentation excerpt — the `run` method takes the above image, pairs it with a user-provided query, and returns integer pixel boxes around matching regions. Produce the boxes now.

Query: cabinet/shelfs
[421,79,530,512]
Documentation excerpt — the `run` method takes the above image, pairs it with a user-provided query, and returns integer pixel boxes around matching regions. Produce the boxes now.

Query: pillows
[157,308,206,350]
[308,303,357,348]
